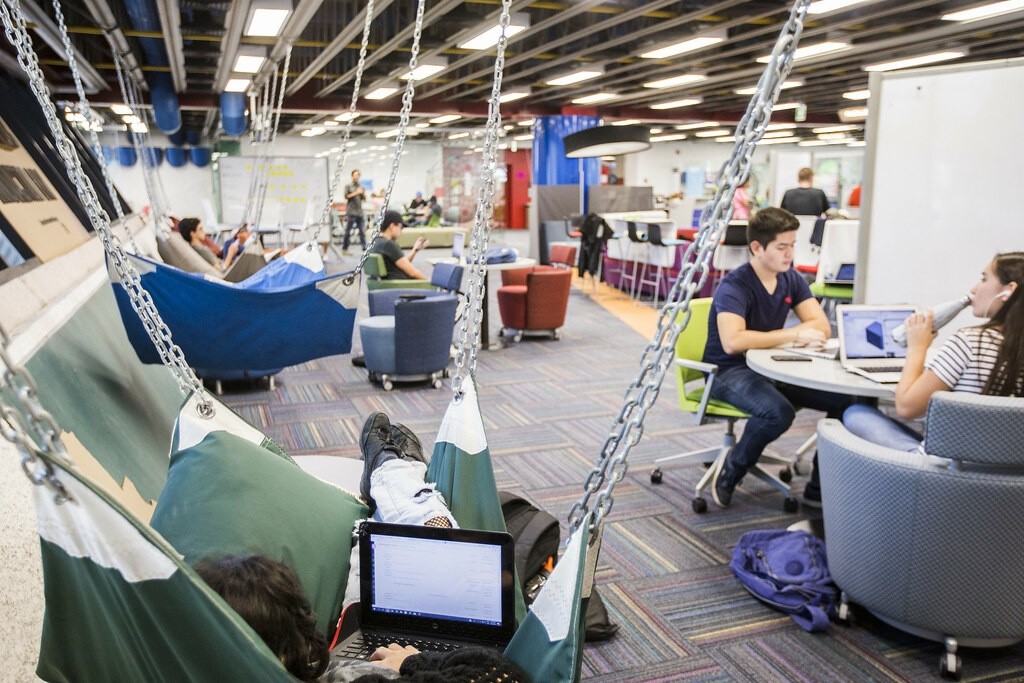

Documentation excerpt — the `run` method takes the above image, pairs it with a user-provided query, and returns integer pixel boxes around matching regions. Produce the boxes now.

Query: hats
[384,211,408,227]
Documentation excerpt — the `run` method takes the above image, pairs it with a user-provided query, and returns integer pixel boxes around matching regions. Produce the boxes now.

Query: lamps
[55,0,1024,166]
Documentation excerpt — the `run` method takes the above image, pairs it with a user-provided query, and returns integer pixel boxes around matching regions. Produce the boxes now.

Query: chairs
[650,294,798,515]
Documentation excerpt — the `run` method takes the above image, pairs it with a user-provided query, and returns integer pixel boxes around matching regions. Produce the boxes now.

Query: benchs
[396,226,472,249]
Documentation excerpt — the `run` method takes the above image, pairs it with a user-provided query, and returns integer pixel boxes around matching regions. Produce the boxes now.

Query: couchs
[815,391,1024,680]
[497,242,576,343]
[362,253,438,292]
[358,263,463,391]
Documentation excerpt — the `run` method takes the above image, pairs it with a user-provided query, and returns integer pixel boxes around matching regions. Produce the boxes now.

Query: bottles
[890,295,972,348]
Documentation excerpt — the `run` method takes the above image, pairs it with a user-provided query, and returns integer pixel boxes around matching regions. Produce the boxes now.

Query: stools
[562,221,750,306]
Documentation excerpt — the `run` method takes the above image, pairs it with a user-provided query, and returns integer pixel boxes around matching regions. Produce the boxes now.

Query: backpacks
[494,491,561,591]
[730,530,840,632]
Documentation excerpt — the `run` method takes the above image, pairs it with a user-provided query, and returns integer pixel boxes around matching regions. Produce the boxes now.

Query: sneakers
[711,447,745,507]
[802,483,822,508]
[359,412,431,503]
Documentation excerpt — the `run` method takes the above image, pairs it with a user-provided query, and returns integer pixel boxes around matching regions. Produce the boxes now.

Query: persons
[374,211,429,281]
[700,207,859,509]
[179,217,300,273]
[400,193,441,226]
[841,251,1024,469]
[191,410,533,683]
[781,167,831,217]
[342,170,367,252]
[732,175,756,221]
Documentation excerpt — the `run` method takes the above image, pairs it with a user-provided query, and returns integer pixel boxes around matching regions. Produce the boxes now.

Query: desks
[808,279,854,327]
[744,336,940,532]
[432,256,536,351]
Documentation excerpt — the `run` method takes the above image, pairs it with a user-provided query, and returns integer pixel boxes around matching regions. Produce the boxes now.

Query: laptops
[328,521,514,664]
[836,264,855,281]
[785,305,923,384]
[427,232,465,265]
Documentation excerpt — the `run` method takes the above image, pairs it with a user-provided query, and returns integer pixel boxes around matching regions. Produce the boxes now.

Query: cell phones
[771,355,812,362]
